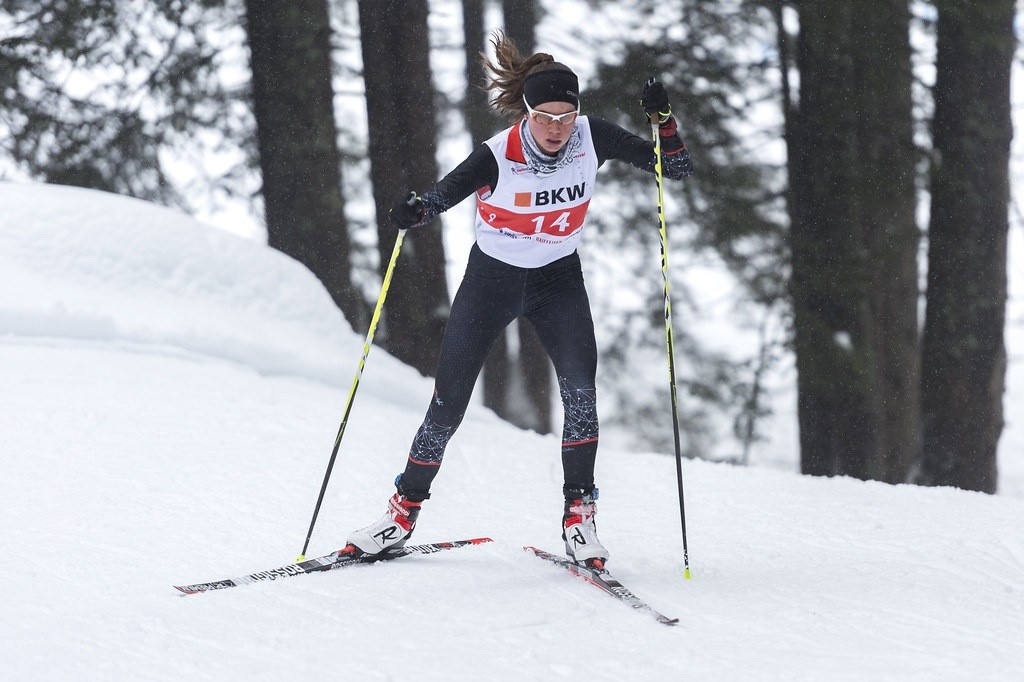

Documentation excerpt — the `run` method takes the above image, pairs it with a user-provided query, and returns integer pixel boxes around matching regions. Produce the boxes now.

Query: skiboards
[173,536,680,625]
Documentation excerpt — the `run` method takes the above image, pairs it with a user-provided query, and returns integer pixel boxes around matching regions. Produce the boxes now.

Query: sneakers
[348,490,422,554]
[564,489,609,561]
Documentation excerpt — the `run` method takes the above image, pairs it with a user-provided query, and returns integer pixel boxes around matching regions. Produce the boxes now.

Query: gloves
[639,82,671,120]
[390,194,423,229]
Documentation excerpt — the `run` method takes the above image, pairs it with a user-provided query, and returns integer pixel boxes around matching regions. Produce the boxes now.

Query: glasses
[523,93,581,125]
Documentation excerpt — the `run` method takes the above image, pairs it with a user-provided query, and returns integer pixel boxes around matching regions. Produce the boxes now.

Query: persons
[338,29,691,571]
